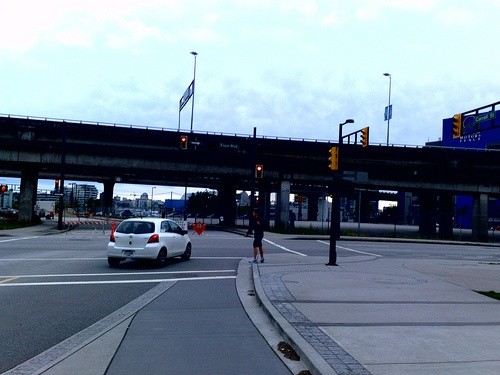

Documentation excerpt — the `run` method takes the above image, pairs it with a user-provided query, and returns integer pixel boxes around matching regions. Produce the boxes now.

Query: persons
[244,208,265,264]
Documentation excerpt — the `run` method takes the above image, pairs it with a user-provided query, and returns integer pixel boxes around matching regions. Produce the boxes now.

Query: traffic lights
[328,147,338,170]
[255,165,263,179]
[180,136,188,149]
[452,113,461,138]
[0,185,8,192]
[54,180,60,190]
[360,128,367,147]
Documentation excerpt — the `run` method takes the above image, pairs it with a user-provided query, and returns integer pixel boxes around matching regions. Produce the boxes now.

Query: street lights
[335,119,355,238]
[382,72,391,146]
[190,51,198,133]
[151,187,156,201]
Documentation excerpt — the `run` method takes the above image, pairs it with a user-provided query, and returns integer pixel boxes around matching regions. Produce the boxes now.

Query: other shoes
[249,259,259,263]
[259,257,264,263]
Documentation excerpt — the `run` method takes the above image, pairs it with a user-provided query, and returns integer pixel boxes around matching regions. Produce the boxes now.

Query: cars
[107,217,192,268]
